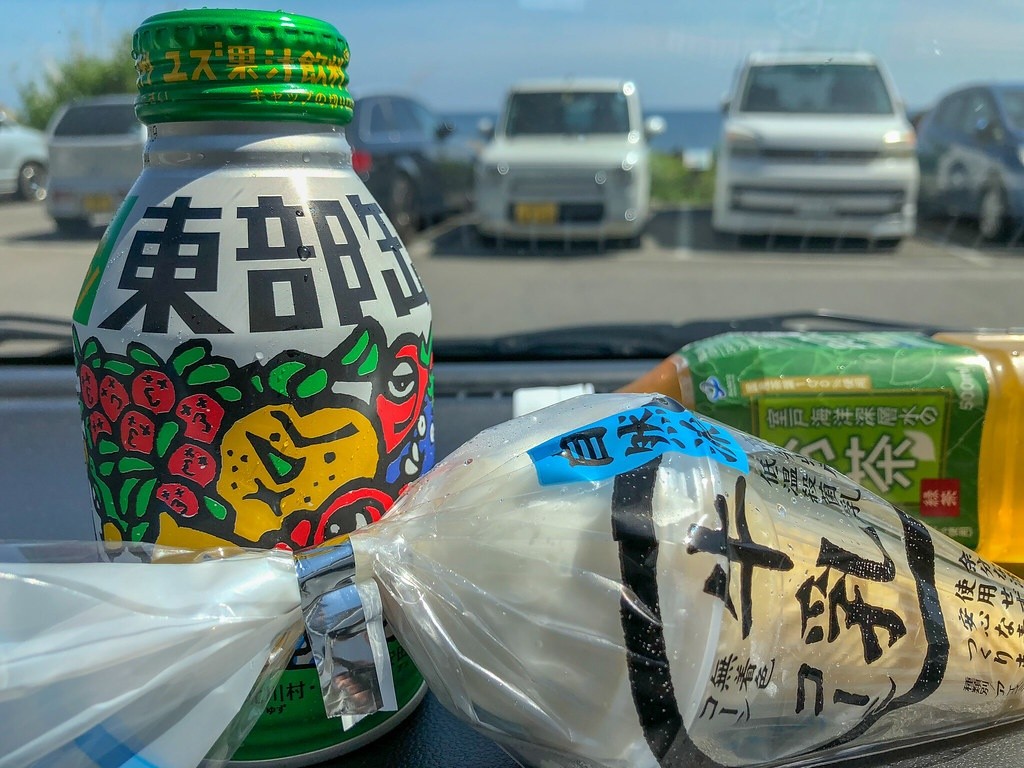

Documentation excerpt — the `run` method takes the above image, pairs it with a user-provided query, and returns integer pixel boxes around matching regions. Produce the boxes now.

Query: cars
[913,82,1024,242]
[342,94,482,240]
[0,111,51,202]
[41,92,151,235]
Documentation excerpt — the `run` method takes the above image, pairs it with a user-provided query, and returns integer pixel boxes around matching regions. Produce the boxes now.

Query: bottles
[513,332,1024,569]
[73,5,436,768]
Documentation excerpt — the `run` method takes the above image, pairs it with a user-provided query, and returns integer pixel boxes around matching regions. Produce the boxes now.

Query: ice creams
[395,423,1023,767]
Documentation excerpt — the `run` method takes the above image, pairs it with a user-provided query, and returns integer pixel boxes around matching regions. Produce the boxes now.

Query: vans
[474,77,667,251]
[709,49,923,251]
